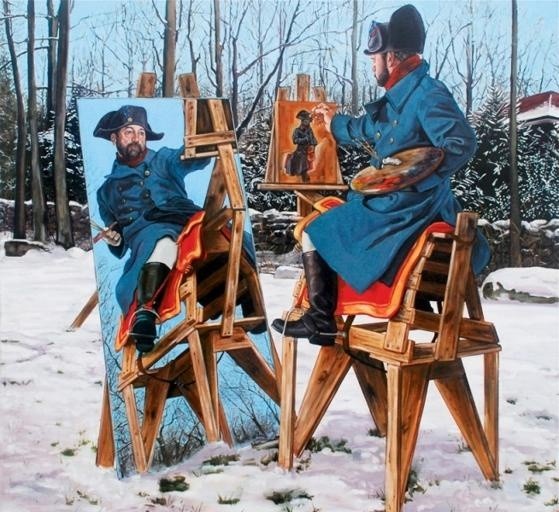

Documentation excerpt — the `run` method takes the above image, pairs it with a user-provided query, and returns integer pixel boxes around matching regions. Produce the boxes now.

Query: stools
[116,207,281,476]
[280,209,500,507]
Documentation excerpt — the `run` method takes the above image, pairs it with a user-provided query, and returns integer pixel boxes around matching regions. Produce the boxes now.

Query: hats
[94,105,164,140]
[363,4,425,54]
[295,111,312,122]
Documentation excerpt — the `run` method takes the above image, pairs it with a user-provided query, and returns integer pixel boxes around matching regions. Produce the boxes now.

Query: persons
[284,110,318,178]
[93,104,267,352]
[269,2,487,348]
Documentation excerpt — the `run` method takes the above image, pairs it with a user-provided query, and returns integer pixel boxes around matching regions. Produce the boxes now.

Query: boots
[132,262,172,352]
[270,251,337,346]
